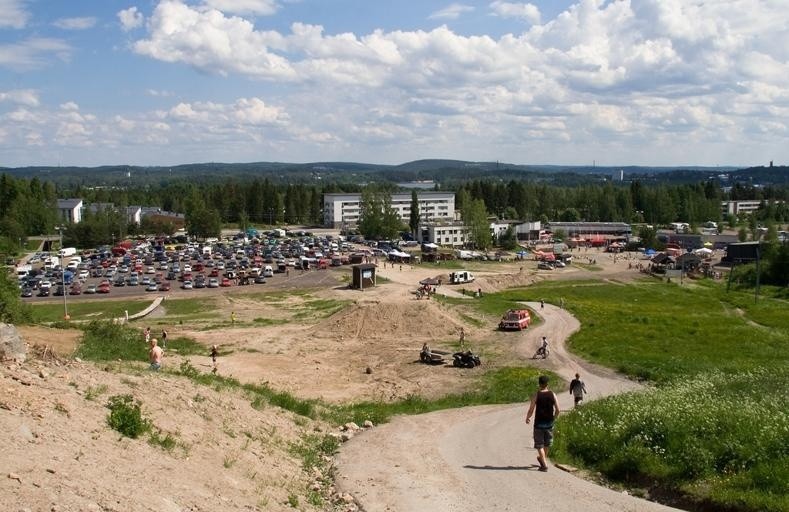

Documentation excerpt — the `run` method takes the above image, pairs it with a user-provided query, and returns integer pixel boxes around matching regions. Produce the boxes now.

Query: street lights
[636,209,644,223]
[282,207,287,226]
[677,237,685,285]
[54,224,67,317]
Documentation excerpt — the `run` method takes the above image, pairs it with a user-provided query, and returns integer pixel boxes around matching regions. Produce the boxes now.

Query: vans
[449,271,474,283]
[393,240,418,247]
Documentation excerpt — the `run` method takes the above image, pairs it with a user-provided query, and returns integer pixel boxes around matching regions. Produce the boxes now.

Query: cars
[440,253,456,261]
[538,260,566,270]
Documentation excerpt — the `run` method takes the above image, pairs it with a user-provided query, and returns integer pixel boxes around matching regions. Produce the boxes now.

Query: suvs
[504,309,530,331]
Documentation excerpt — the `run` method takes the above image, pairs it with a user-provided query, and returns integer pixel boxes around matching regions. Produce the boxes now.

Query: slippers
[537,456,548,471]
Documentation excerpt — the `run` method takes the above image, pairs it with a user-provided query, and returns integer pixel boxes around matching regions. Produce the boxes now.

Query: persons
[231,311,237,323]
[421,343,428,363]
[459,327,465,345]
[209,345,219,362]
[542,337,548,359]
[462,287,465,296]
[143,326,167,372]
[541,299,544,309]
[526,376,560,471]
[570,373,587,408]
